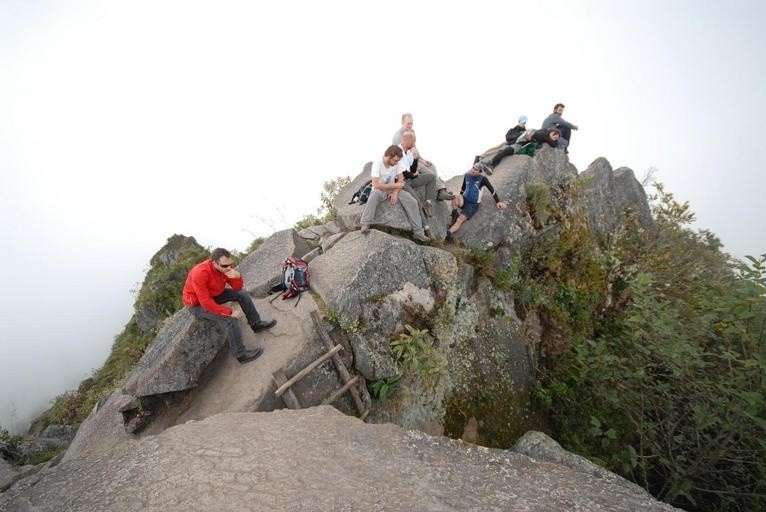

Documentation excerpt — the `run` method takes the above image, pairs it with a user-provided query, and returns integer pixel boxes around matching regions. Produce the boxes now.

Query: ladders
[271,309,370,424]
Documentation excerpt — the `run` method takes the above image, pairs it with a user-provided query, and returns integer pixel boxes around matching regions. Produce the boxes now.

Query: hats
[518,115,528,124]
[472,162,483,170]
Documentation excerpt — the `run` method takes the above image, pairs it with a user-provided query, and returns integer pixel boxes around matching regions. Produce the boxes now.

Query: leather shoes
[237,347,264,366]
[251,319,277,333]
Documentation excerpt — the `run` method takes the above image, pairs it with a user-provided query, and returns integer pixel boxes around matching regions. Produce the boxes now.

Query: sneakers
[422,201,433,218]
[360,225,370,234]
[412,232,431,243]
[450,209,459,225]
[436,192,456,201]
[424,229,436,242]
[446,230,455,242]
[474,156,494,175]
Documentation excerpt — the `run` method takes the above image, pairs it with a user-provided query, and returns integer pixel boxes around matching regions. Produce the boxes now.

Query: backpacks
[267,255,310,308]
[347,180,372,205]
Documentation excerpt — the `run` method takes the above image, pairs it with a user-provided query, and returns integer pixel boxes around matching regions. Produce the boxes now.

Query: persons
[360,103,578,242]
[183,247,278,365]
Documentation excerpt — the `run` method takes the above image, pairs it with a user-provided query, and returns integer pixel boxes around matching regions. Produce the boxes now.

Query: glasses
[219,264,232,268]
